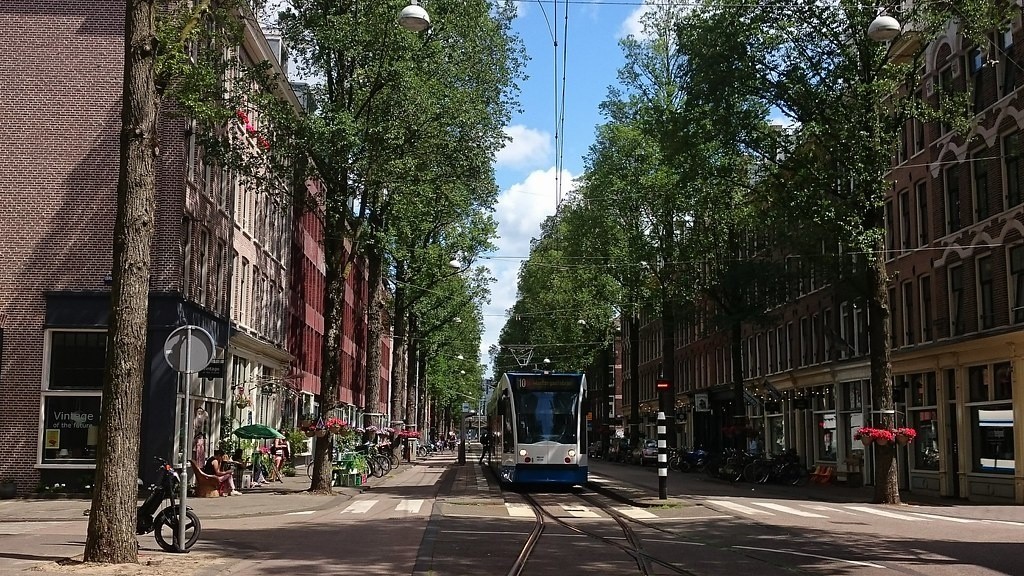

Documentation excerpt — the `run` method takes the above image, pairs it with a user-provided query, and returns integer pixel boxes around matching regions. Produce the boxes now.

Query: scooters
[135,456,202,552]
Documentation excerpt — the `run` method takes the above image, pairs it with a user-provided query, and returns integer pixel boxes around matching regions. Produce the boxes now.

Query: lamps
[867,6,901,41]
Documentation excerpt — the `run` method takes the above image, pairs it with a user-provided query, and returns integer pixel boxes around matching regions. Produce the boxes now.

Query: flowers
[398,431,421,438]
[308,426,316,430]
[326,418,346,427]
[852,426,918,444]
[356,426,396,436]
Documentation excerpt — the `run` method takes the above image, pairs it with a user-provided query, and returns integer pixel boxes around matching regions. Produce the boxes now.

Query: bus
[486,368,592,486]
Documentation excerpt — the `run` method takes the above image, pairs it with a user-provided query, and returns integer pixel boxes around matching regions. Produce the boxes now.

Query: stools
[341,473,356,487]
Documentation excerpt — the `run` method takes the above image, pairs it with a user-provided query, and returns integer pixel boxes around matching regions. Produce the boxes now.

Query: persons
[440,432,445,452]
[274,427,287,479]
[747,434,757,450]
[204,450,242,496]
[449,432,455,450]
[232,449,246,468]
[479,432,490,464]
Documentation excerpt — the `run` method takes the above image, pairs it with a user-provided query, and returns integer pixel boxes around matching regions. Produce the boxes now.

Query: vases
[897,435,908,446]
[876,438,888,446]
[860,436,873,446]
[330,425,341,433]
[355,431,363,436]
[305,430,314,437]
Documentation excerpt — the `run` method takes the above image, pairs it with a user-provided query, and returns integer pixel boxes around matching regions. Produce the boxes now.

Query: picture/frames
[378,434,386,438]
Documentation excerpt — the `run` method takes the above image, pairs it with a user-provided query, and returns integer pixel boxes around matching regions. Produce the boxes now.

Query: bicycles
[306,459,314,481]
[667,445,802,485]
[333,442,399,478]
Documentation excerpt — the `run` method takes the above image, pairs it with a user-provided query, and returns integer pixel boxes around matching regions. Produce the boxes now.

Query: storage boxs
[356,476,361,485]
[362,474,367,482]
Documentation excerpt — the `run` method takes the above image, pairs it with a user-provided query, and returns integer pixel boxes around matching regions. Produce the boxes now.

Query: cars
[589,440,602,458]
[632,438,657,465]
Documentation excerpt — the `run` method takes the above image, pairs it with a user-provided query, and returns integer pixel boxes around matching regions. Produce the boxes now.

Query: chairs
[189,458,224,497]
[808,463,834,487]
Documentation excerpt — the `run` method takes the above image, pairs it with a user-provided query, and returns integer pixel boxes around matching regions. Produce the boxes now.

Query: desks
[836,471,861,488]
[332,459,355,485]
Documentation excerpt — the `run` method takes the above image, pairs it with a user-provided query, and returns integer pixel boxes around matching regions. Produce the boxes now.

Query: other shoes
[231,490,242,496]
[261,479,270,484]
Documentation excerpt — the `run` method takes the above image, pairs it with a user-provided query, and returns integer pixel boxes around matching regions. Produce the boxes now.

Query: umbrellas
[234,424,286,482]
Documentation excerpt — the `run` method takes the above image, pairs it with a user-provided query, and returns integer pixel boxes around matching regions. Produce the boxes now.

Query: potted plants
[233,397,253,409]
[314,424,327,438]
